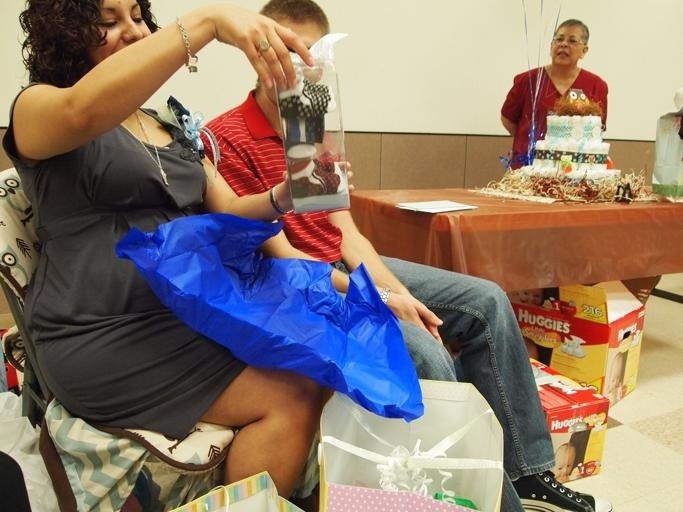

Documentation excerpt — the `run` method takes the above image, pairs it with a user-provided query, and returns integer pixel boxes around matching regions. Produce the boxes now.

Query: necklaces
[120,111,172,187]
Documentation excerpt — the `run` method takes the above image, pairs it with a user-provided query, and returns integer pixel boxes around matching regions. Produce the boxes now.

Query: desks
[346,188,683,303]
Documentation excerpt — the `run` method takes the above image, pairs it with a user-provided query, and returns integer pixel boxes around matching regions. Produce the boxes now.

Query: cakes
[519,89,621,190]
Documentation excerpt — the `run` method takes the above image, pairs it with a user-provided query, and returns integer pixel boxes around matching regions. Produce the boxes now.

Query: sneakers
[510,471,613,512]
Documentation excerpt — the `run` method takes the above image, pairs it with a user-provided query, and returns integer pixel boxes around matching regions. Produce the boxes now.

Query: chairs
[0,166,318,512]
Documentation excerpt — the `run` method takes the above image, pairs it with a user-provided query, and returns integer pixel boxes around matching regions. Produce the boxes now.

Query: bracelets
[171,18,198,72]
[375,287,390,304]
[268,187,294,214]
[1,0,363,501]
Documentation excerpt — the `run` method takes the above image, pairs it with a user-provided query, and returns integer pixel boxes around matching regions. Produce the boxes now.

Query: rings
[256,38,269,56]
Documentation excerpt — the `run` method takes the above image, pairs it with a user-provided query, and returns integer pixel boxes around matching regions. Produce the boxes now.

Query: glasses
[553,37,585,45]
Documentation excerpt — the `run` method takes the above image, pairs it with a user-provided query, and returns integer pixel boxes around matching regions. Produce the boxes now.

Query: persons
[508,285,543,311]
[501,18,610,180]
[194,0,601,511]
[548,443,576,483]
[606,351,625,403]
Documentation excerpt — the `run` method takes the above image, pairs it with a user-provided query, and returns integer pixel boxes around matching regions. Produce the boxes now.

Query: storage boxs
[506,275,660,483]
